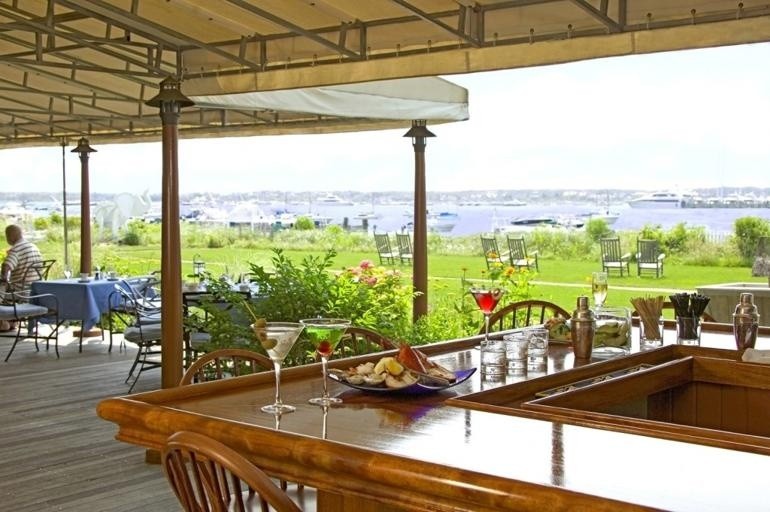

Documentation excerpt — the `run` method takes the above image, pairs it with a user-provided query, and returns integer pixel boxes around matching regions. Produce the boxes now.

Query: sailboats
[574,191,620,225]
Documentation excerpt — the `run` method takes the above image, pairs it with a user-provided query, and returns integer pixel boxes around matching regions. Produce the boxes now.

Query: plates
[320,367,421,397]
[407,367,477,393]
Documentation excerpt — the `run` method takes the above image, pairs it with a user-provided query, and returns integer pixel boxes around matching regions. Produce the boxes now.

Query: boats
[485,214,616,235]
[400,207,459,233]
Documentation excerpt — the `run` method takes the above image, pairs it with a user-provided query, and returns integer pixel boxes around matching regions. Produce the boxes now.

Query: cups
[591,272,609,308]
[502,334,531,360]
[638,315,666,346]
[480,339,507,366]
[525,328,550,355]
[63,264,118,284]
[675,316,702,346]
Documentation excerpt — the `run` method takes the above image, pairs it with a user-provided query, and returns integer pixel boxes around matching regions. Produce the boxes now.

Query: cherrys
[318,340,332,355]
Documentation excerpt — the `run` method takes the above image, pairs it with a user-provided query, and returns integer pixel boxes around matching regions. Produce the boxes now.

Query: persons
[0,225,47,334]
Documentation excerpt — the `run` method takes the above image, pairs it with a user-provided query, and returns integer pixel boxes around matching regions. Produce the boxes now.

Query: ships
[316,195,355,206]
[626,191,702,210]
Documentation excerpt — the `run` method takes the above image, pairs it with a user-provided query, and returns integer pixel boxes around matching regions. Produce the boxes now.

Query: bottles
[570,296,595,360]
[732,293,760,351]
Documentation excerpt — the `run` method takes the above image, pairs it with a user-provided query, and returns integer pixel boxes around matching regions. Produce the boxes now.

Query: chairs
[1,258,251,396]
[599,235,665,279]
[373,232,414,267]
[480,234,539,274]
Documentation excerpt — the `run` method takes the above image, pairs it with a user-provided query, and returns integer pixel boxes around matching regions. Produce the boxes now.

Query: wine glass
[300,317,352,406]
[470,287,505,350]
[250,320,305,414]
